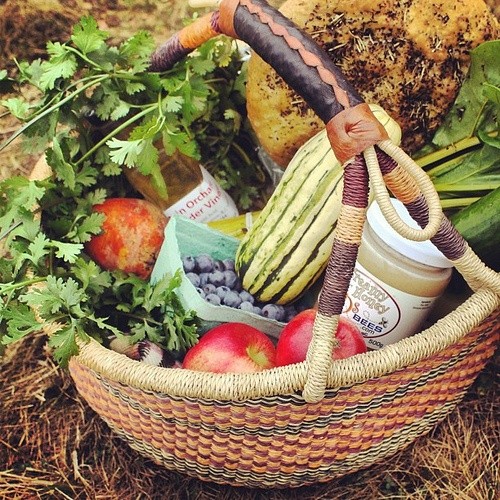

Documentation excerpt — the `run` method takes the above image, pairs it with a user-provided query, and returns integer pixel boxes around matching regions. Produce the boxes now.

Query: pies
[244,0,500,171]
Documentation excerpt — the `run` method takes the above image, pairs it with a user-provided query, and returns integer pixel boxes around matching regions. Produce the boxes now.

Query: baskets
[22,1,500,487]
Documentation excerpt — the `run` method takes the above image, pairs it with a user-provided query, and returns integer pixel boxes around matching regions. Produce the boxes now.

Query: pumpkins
[235,104,401,305]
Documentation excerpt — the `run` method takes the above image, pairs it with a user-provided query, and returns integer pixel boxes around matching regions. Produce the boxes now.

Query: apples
[276,309,368,367]
[181,323,276,374]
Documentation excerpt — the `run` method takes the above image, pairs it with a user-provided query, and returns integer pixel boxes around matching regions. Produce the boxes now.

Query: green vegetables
[0,15,266,370]
[429,40,500,253]
